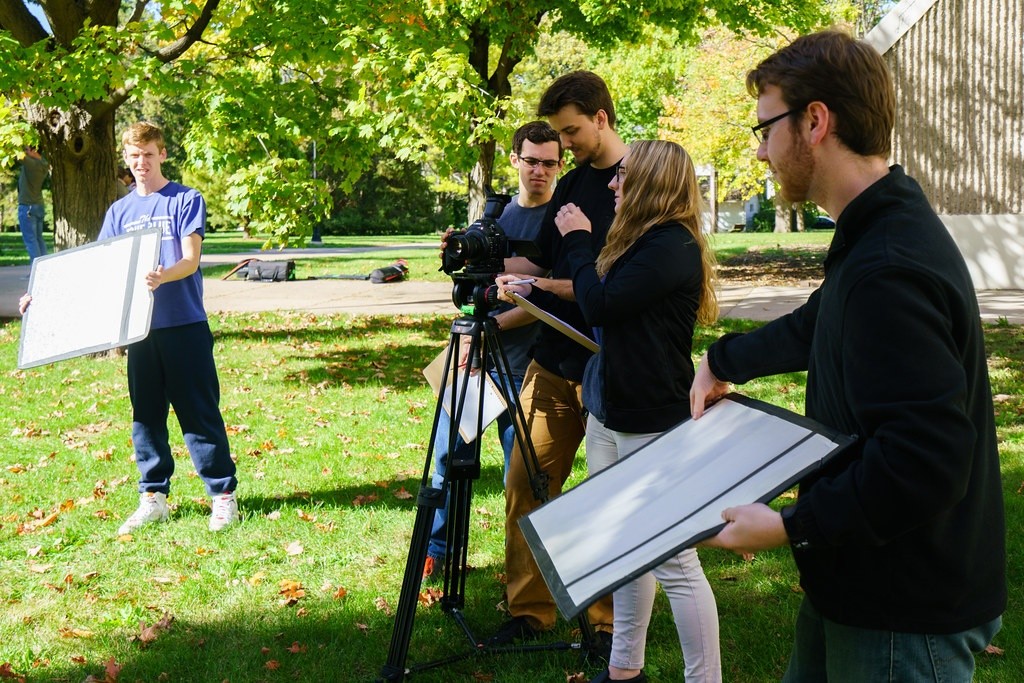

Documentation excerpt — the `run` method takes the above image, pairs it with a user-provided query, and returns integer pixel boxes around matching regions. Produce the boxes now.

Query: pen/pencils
[504,277,538,285]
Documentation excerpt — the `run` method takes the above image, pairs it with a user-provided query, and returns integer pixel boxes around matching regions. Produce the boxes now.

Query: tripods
[382,271,604,683]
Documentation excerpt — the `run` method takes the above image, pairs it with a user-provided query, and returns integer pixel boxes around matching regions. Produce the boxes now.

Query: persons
[19,122,238,536]
[18,144,51,279]
[687,31,1009,683]
[421,70,722,683]
[117,167,138,200]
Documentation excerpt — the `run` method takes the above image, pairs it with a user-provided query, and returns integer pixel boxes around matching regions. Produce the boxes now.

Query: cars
[753,209,835,232]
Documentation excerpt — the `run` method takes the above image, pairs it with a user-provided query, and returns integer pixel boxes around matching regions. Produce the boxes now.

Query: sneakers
[210,491,238,532]
[118,492,168,537]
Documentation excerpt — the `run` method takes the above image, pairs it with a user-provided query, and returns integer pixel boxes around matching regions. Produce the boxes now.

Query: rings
[563,210,571,216]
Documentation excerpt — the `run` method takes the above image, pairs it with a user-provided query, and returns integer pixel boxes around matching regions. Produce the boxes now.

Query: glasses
[751,102,811,145]
[616,165,626,182]
[518,154,561,170]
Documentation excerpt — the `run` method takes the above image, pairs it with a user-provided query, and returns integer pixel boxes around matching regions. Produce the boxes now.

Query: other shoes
[590,667,647,683]
[422,557,444,587]
[494,619,539,645]
[577,631,613,665]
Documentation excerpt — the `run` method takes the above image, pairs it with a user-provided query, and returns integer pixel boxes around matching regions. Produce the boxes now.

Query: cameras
[440,192,516,274]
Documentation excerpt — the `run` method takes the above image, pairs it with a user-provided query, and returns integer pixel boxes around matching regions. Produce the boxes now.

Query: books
[422,334,509,444]
